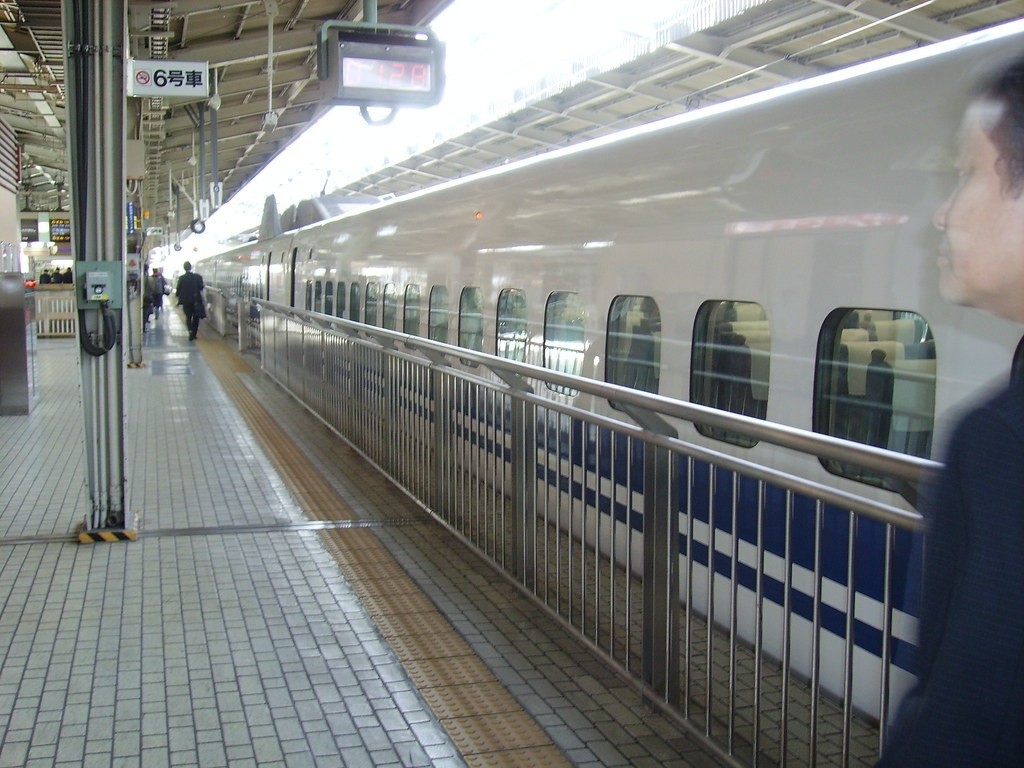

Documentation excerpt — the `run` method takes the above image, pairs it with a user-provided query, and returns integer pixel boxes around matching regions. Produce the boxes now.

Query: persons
[40,267,72,284]
[873,58,1024,768]
[143,264,170,334]
[175,262,206,341]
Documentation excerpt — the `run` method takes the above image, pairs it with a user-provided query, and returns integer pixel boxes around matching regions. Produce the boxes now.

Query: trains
[173,17,1024,728]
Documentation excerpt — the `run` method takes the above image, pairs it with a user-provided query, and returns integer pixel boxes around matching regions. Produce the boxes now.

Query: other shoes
[155,316,159,320]
[189,333,197,340]
[147,320,150,323]
[144,330,147,333]
